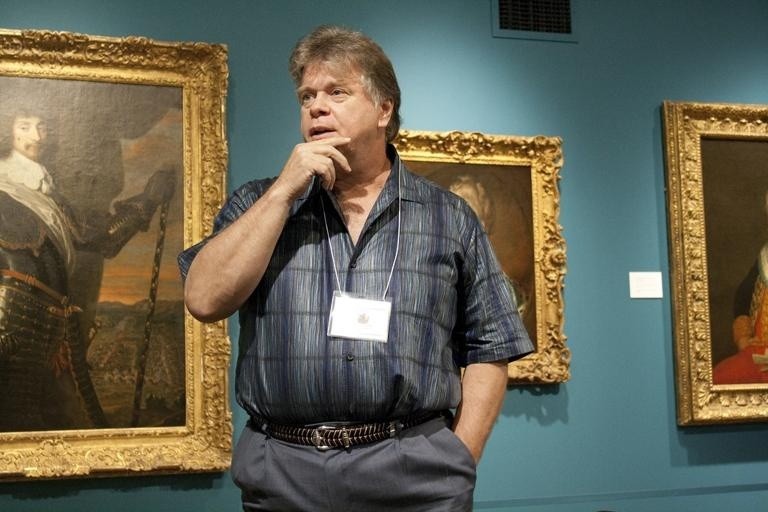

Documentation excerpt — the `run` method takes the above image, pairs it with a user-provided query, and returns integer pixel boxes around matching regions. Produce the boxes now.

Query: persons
[0,98,174,429]
[711,242,768,382]
[178,23,537,510]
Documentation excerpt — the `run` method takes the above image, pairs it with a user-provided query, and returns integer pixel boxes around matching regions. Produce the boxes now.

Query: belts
[1,269,73,307]
[250,410,441,450]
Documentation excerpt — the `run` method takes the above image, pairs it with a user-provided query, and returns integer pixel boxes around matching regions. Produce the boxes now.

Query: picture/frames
[662,99,768,430]
[0,28,233,481]
[391,130,570,388]
